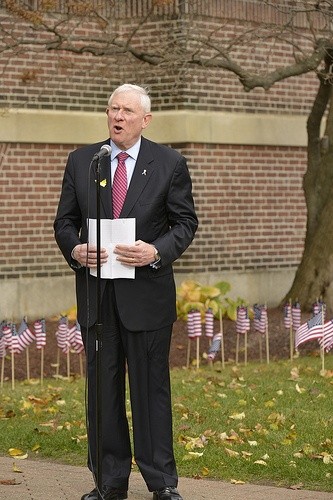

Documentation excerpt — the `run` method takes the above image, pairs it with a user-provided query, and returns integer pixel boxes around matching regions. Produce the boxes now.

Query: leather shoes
[153,485,182,500]
[81,487,127,500]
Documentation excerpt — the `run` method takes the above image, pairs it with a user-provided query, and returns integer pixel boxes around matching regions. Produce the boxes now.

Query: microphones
[91,145,112,162]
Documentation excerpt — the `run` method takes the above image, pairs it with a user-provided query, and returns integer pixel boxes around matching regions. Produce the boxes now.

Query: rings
[133,258,134,262]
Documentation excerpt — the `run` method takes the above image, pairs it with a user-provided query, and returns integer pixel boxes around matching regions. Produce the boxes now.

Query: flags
[0,319,47,358]
[187,308,222,361]
[54,317,85,354]
[284,302,333,353]
[236,303,267,335]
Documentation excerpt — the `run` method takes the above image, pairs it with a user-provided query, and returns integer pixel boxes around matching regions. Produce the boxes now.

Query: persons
[53,84,198,500]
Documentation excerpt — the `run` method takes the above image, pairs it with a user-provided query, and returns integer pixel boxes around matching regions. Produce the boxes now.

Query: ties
[112,152,131,220]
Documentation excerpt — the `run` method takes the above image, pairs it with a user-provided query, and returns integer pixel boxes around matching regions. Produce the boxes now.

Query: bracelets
[155,249,159,260]
